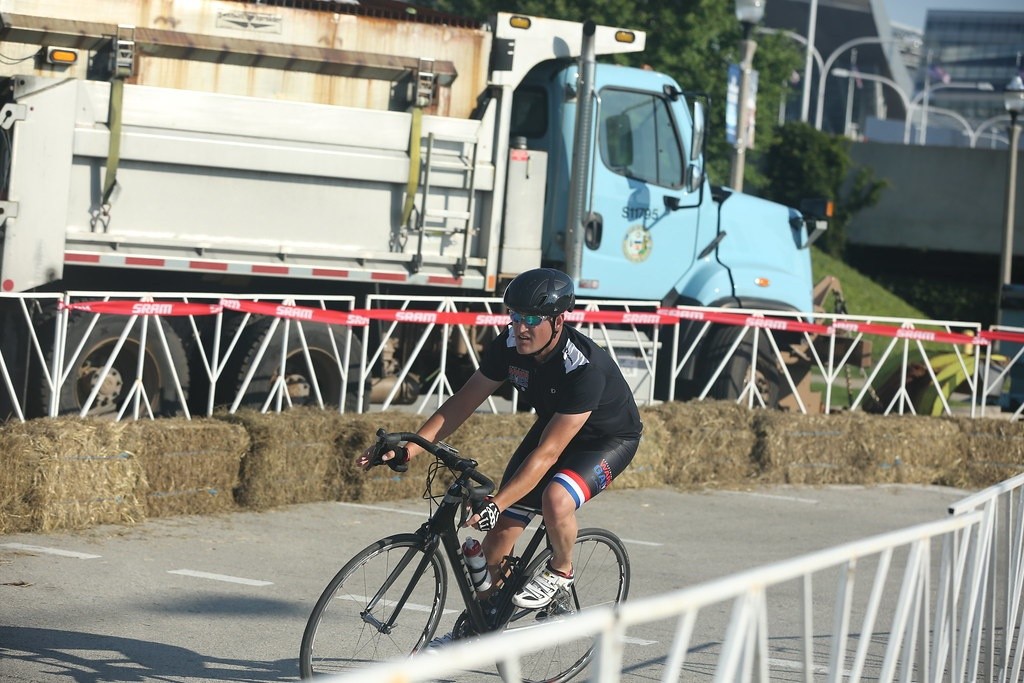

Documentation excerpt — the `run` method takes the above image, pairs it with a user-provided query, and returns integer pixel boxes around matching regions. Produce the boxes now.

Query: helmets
[503,268,575,318]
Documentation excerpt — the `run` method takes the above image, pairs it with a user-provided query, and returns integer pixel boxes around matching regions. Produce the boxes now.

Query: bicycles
[298,427,629,681]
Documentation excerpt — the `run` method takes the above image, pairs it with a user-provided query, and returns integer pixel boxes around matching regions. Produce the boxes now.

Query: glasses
[509,310,550,326]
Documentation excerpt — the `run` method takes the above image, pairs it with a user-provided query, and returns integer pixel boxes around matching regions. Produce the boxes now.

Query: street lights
[996,77,1024,353]
[728,1,766,192]
[757,28,1024,149]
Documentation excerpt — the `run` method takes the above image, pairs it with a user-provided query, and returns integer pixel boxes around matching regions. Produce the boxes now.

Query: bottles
[462,536,493,592]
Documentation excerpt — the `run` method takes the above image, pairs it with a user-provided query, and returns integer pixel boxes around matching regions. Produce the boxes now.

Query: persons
[355,267,643,609]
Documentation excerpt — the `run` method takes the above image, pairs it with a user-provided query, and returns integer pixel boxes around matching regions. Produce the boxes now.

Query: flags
[928,62,951,85]
[788,69,800,87]
[854,64,863,89]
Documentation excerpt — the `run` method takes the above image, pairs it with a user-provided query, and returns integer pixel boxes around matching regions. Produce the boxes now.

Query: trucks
[0,1,818,417]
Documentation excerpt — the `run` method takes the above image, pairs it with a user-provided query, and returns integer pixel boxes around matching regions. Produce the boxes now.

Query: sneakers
[511,559,574,608]
[425,631,454,653]
